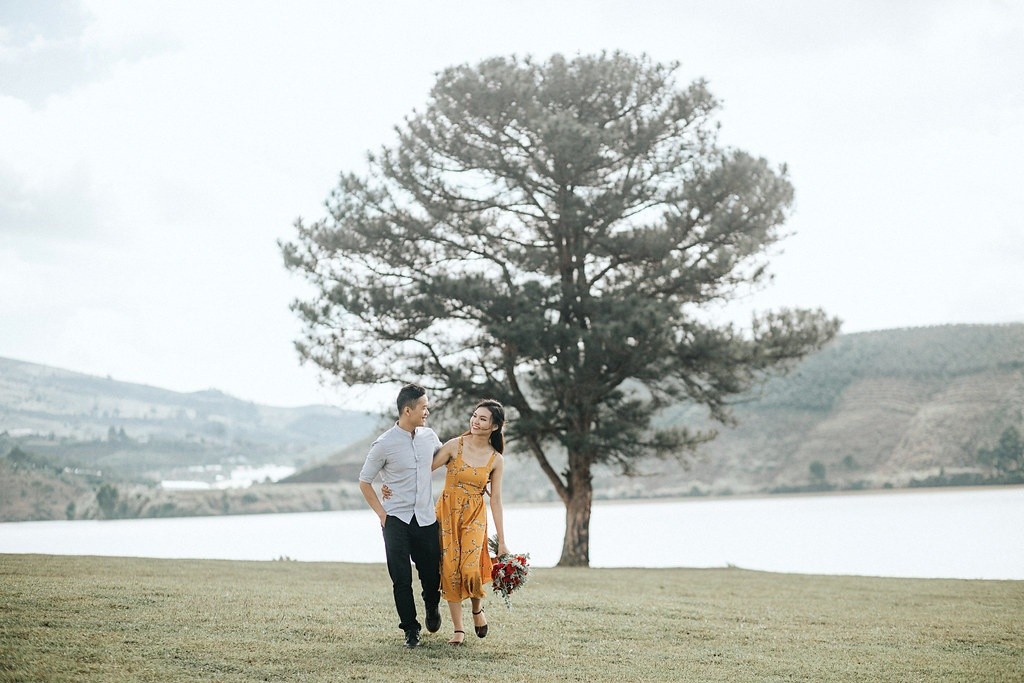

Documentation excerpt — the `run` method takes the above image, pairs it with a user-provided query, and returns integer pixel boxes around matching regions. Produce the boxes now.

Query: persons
[359,384,491,649]
[381,400,509,645]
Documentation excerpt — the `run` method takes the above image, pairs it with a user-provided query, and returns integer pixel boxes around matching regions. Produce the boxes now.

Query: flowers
[488,534,535,613]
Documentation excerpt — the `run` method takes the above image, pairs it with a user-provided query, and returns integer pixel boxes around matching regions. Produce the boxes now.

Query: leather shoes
[403,632,421,649]
[425,606,441,633]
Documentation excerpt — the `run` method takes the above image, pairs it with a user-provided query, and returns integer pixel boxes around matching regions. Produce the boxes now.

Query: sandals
[447,630,465,648]
[472,609,488,638]
